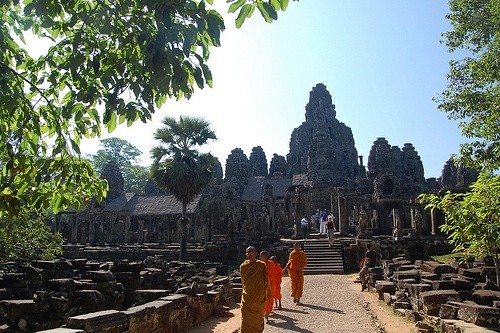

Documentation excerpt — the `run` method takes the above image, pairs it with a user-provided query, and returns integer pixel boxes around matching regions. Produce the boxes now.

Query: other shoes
[329,246,331,248]
[332,245,334,246]
[361,286,368,292]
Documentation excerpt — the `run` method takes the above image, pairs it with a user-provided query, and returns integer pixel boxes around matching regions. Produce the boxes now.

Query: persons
[359,244,377,292]
[301,214,308,238]
[259,250,282,323]
[240,246,269,333]
[325,217,334,248]
[311,208,336,236]
[287,242,307,305]
